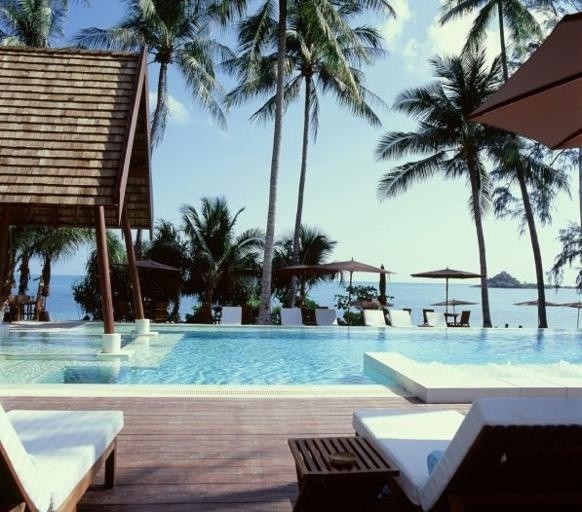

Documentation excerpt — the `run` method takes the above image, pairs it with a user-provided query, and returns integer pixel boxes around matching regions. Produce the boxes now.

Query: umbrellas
[313,256,399,302]
[376,263,388,306]
[463,10,581,152]
[273,262,335,320]
[557,300,582,328]
[510,298,561,326]
[112,258,182,279]
[428,296,480,315]
[408,266,486,313]
[348,297,394,310]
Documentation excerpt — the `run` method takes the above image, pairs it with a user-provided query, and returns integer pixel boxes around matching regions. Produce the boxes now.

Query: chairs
[352,397,582,512]
[0,401,124,512]
[220,306,471,327]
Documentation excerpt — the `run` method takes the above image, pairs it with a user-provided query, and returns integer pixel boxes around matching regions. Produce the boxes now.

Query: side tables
[289,436,400,512]
